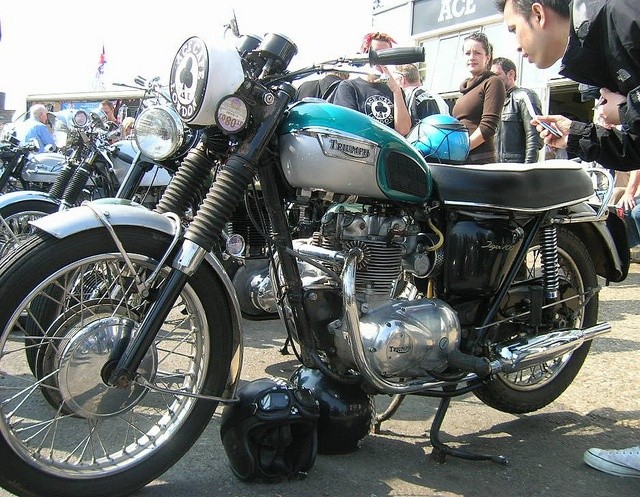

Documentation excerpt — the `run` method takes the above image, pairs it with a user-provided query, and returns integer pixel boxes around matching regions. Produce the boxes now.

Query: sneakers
[583,443,640,477]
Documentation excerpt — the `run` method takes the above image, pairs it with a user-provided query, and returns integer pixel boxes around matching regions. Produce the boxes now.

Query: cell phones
[526,98,562,139]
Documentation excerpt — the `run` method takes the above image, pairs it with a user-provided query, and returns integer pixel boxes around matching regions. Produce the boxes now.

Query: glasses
[251,384,321,421]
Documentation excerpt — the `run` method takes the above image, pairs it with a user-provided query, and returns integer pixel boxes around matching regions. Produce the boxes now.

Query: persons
[395,63,439,125]
[334,33,411,136]
[495,1,640,481]
[293,71,350,100]
[100,100,124,144]
[492,56,542,162]
[124,117,135,138]
[615,171,640,261]
[452,30,506,166]
[16,106,56,152]
[416,59,450,115]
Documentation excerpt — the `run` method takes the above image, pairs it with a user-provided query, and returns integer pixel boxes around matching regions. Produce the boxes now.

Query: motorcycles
[24,76,292,379]
[0,102,172,333]
[0,131,107,248]
[0,8,630,497]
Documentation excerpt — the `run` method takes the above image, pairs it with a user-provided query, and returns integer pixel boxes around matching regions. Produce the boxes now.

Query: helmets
[291,364,375,452]
[403,114,470,164]
[222,376,318,482]
